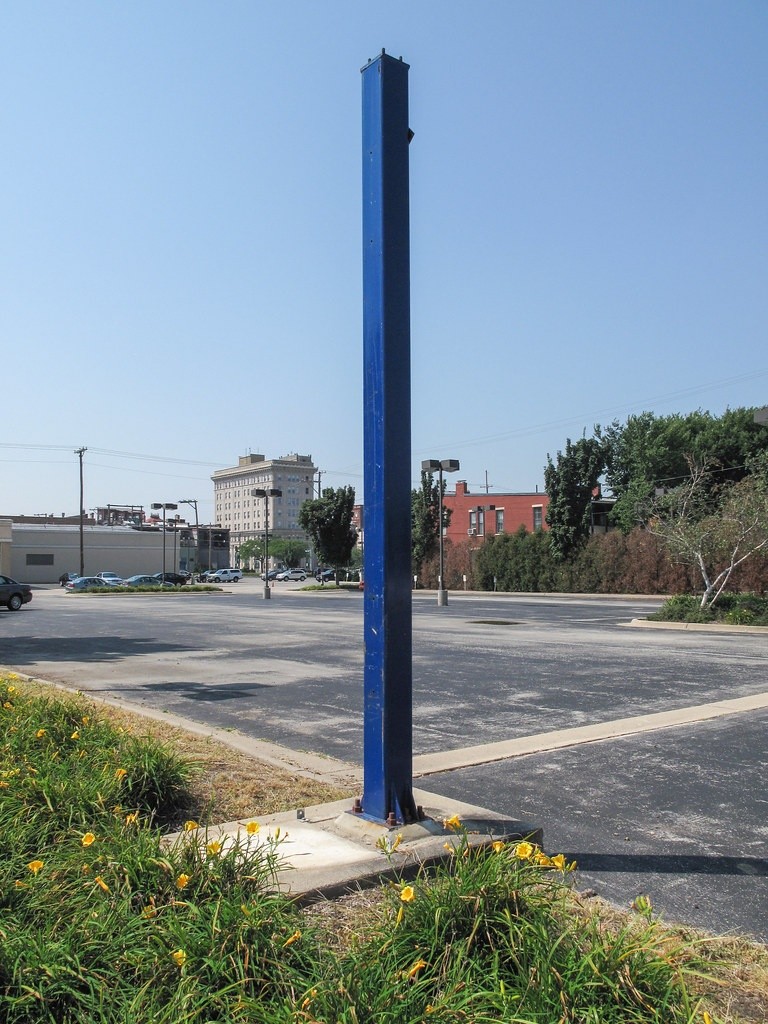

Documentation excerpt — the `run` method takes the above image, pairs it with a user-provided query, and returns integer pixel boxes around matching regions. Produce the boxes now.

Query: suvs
[194,570,216,583]
[276,570,307,582]
[206,568,243,583]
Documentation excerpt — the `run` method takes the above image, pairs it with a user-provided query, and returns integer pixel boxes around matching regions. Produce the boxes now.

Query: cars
[0,575,32,610]
[122,575,176,587]
[58,573,80,587]
[316,569,353,583]
[153,572,187,588]
[94,572,123,585]
[260,570,277,577]
[314,569,330,578]
[65,577,119,593]
[179,569,192,581]
[261,570,286,581]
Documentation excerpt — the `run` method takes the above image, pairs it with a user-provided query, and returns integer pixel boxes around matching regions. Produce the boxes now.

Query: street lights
[251,488,283,600]
[472,504,496,543]
[420,460,459,606]
[150,503,177,580]
